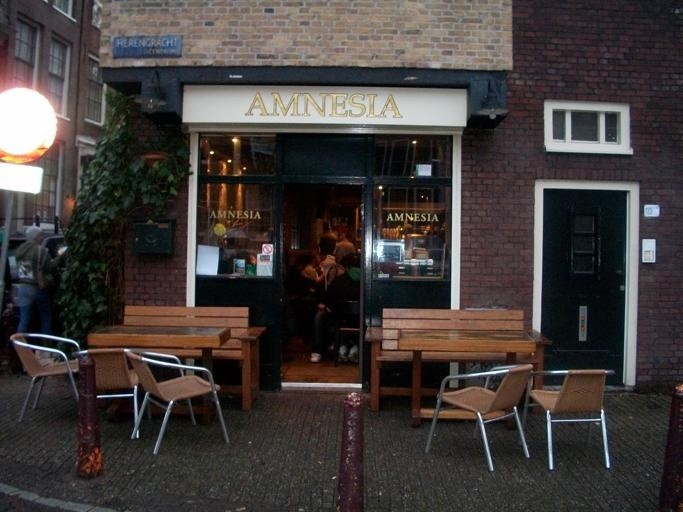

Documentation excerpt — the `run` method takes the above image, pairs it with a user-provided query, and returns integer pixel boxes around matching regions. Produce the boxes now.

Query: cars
[204,223,279,272]
[0,230,67,352]
[372,229,445,276]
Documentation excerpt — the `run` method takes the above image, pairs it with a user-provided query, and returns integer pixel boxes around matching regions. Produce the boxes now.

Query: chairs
[71,348,141,439]
[333,300,360,368]
[124,348,229,455]
[522,368,615,471]
[423,362,534,472]
[10,332,83,421]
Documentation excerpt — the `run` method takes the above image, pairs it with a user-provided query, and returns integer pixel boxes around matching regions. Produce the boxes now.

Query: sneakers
[311,352,321,362]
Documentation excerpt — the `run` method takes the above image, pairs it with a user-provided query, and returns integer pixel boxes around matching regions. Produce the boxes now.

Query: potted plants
[54,85,191,350]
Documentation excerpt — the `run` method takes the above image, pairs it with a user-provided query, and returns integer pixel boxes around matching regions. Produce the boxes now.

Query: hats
[26,226,41,239]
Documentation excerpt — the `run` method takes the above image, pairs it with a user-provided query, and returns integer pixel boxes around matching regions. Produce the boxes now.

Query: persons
[10,224,57,365]
[0,224,4,259]
[280,228,360,367]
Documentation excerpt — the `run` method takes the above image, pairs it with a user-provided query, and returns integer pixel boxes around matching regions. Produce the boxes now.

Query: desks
[87,325,231,424]
[398,328,536,429]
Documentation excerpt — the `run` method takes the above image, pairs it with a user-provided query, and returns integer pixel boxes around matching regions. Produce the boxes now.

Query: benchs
[123,305,267,411]
[365,307,551,426]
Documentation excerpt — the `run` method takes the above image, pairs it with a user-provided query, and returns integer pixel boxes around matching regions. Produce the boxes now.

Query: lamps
[477,79,508,119]
[133,70,168,110]
